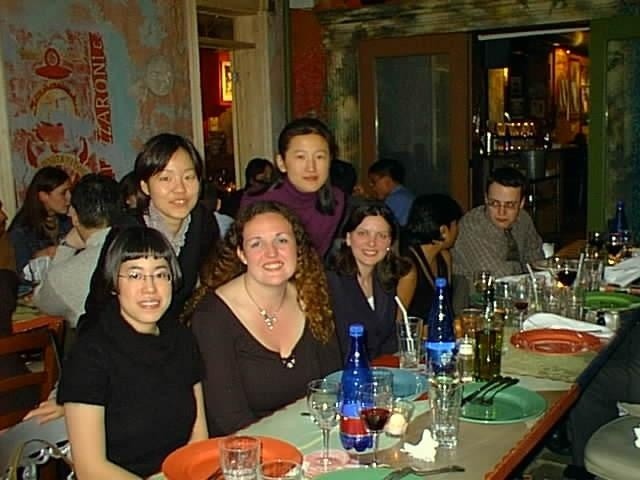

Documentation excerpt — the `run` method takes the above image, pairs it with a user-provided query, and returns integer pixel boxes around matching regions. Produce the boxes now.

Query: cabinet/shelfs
[476,148,565,242]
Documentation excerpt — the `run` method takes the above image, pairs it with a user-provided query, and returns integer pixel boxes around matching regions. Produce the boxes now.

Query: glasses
[119,268,172,283]
[479,201,514,210]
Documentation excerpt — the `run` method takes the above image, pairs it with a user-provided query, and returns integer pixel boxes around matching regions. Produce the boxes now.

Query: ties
[503,227,520,262]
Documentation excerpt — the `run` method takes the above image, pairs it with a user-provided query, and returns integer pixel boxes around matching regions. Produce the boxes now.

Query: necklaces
[360,273,373,300]
[240,271,289,331]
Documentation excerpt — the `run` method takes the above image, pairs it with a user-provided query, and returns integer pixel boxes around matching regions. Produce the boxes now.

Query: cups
[396,316,424,369]
[218,434,304,480]
[385,375,464,449]
[525,259,621,331]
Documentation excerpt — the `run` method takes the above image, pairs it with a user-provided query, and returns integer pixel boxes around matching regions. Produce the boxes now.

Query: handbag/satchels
[2,439,76,480]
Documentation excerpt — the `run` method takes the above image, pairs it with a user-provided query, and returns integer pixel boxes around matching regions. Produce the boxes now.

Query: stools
[585,412,640,480]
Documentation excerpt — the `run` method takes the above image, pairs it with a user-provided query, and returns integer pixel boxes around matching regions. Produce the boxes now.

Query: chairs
[519,150,544,227]
[0,326,61,479]
[0,232,18,272]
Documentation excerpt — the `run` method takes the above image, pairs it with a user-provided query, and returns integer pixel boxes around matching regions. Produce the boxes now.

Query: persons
[0,200,17,340]
[392,192,466,339]
[21,133,235,426]
[354,158,416,226]
[27,172,126,330]
[55,225,210,480]
[120,149,354,238]
[189,201,345,436]
[324,198,401,368]
[234,118,346,260]
[447,168,545,319]
[7,165,74,285]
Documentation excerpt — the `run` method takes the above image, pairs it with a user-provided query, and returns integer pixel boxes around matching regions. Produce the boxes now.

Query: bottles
[337,324,376,451]
[423,275,505,382]
[610,200,632,258]
[484,119,537,153]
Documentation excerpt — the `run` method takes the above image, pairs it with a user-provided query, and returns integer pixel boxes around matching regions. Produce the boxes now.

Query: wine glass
[587,230,624,267]
[304,367,394,470]
[472,268,529,334]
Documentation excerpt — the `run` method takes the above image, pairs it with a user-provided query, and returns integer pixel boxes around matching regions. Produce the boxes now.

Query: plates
[531,258,640,313]
[161,434,424,480]
[321,366,548,426]
[509,328,602,356]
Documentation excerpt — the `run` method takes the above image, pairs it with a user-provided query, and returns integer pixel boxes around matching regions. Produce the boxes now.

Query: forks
[384,464,466,480]
[471,376,521,406]
